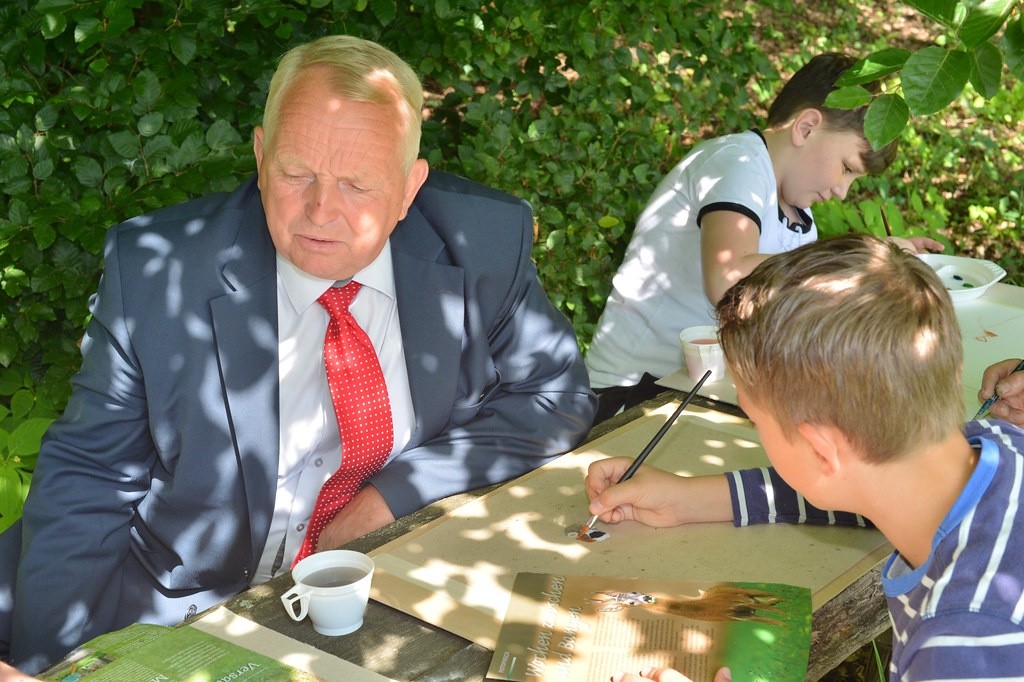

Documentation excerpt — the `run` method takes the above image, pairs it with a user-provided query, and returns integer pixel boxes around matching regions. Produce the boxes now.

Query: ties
[290,280,394,571]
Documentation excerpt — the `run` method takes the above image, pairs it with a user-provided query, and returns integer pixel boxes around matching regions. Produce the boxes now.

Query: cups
[680,325,726,382]
[280,549,375,636]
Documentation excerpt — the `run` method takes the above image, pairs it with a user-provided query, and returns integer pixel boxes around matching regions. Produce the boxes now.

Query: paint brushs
[877,203,897,237]
[968,359,1024,420]
[577,369,714,538]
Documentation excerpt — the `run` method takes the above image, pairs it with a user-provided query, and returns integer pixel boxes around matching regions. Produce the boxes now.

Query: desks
[33,272,1024,682]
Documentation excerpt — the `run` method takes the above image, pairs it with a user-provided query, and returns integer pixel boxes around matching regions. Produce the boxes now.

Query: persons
[585,231,1024,682]
[584,52,945,425]
[0,35,600,678]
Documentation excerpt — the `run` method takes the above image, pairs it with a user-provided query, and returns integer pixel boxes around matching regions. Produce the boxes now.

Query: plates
[915,254,1006,300]
